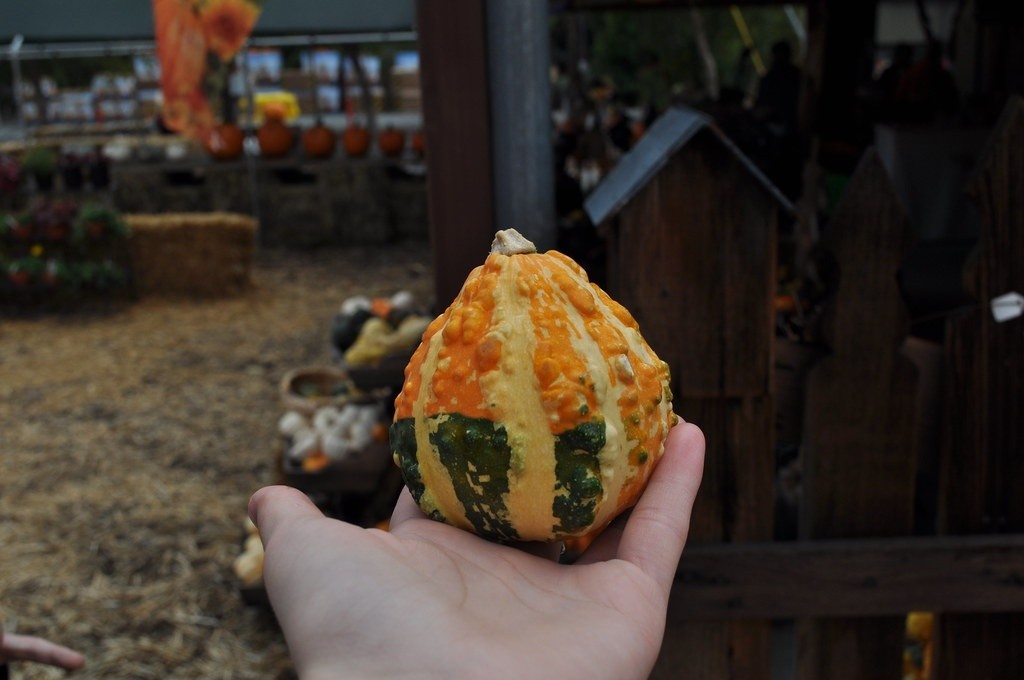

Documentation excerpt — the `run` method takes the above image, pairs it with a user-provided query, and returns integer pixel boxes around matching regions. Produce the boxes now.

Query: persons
[242,410,708,680]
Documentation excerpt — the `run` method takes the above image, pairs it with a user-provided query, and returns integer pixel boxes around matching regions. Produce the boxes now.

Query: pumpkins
[388,225,680,545]
[208,117,425,161]
